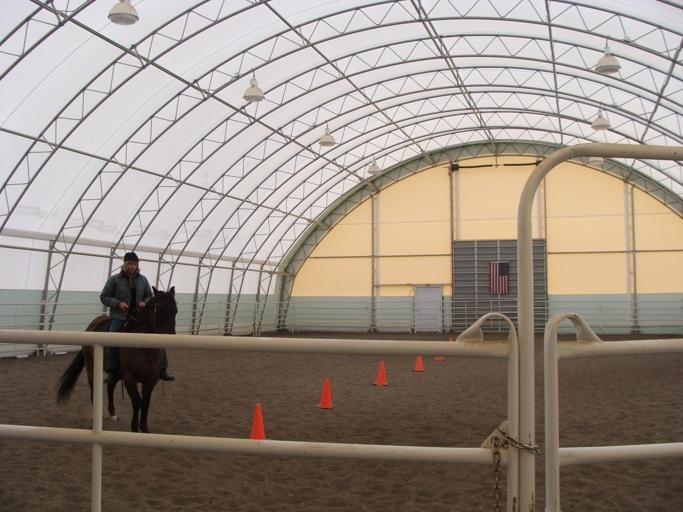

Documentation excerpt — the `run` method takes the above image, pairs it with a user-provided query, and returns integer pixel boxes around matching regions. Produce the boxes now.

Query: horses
[54,285,178,434]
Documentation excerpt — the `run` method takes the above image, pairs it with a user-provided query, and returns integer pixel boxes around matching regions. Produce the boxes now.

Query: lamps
[108,0,139,25]
[592,106,609,130]
[368,155,381,173]
[596,38,621,74]
[319,121,337,146]
[243,68,265,102]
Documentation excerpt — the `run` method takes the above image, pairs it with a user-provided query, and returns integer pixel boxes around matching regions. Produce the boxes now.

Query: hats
[123,253,139,261]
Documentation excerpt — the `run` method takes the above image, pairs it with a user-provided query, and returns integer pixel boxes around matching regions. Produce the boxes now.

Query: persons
[99,252,175,383]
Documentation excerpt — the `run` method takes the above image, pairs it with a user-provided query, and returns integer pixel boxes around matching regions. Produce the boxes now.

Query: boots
[159,368,173,381]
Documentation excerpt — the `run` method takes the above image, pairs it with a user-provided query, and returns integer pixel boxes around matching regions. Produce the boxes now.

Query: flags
[490,262,509,294]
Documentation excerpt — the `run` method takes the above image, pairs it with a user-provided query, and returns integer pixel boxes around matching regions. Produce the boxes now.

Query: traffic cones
[434,356,445,361]
[372,360,387,386]
[248,402,266,440]
[315,378,336,409]
[448,336,452,341]
[412,355,424,372]
